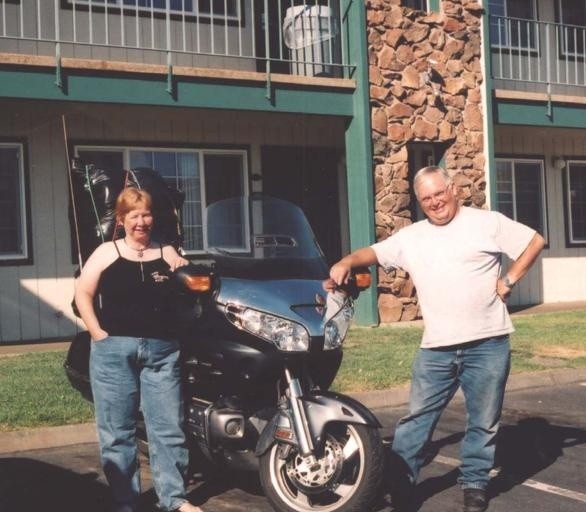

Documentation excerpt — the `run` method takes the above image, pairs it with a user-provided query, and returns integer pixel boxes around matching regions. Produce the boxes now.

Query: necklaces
[124,236,153,282]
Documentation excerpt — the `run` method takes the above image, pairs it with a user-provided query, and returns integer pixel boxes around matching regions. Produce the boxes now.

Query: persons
[74,188,203,512]
[329,166,545,512]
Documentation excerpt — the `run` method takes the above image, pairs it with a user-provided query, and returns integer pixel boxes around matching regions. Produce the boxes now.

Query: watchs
[504,274,513,289]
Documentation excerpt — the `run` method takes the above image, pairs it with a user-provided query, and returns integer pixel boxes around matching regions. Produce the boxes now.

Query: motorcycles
[62,194,387,512]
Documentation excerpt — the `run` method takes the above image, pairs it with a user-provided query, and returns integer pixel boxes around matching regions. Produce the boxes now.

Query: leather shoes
[463,485,487,512]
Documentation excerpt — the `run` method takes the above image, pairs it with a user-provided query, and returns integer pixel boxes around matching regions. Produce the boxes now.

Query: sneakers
[178,502,203,512]
